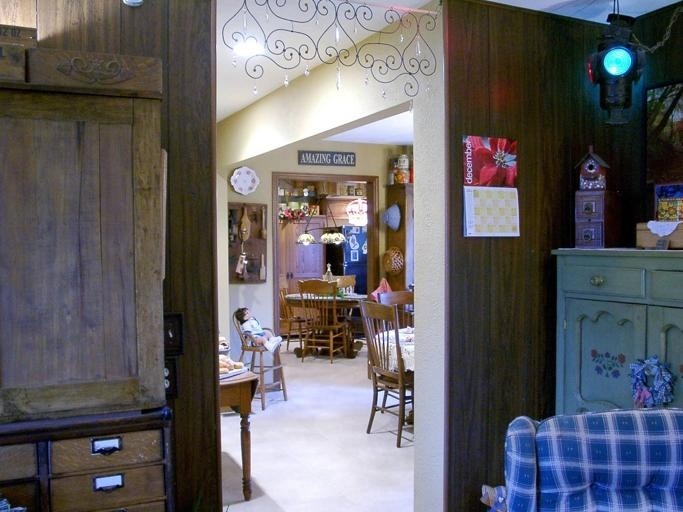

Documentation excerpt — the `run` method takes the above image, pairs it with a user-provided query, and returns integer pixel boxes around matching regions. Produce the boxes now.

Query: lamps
[298,193,347,246]
[346,199,368,226]
[586,0,683,127]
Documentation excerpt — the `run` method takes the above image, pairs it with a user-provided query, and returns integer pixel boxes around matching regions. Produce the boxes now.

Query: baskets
[382,247,404,277]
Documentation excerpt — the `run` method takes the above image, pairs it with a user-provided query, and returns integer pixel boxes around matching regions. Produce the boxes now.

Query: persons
[234,307,282,352]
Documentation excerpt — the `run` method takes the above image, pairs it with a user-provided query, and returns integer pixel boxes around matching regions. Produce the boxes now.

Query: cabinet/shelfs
[385,183,414,327]
[550,247,683,416]
[277,214,327,336]
[1,406,177,512]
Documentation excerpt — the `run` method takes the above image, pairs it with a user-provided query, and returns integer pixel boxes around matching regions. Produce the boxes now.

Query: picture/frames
[640,79,683,191]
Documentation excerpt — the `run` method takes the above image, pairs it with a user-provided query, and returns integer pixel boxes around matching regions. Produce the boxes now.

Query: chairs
[480,407,683,512]
[233,274,414,447]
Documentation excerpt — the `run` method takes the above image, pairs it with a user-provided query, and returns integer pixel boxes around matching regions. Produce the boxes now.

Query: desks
[219,370,259,500]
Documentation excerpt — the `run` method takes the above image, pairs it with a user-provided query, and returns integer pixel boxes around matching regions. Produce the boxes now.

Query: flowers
[279,207,304,223]
